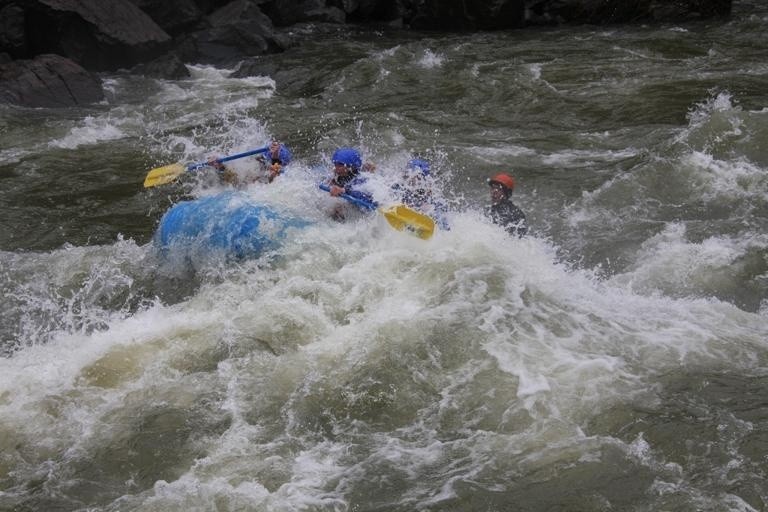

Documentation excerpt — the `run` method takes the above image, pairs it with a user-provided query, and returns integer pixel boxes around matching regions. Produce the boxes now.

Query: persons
[320,147,373,209]
[487,174,526,238]
[207,141,290,190]
[392,159,448,230]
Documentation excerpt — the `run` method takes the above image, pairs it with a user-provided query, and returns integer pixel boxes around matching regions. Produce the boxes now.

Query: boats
[158,192,318,265]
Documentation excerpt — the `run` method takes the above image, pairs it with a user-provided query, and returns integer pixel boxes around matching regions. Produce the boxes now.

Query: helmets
[407,159,429,177]
[332,149,361,169]
[255,144,289,166]
[487,175,514,192]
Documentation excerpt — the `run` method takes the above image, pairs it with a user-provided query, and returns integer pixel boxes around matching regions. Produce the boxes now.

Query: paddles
[143,145,271,187]
[278,170,436,238]
